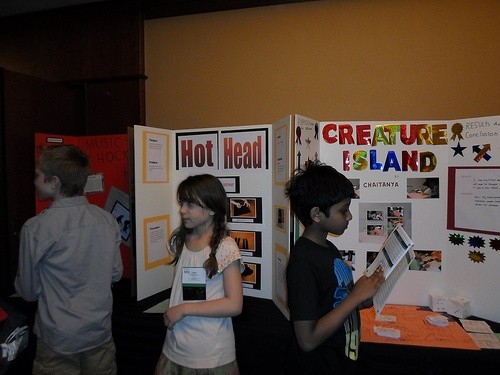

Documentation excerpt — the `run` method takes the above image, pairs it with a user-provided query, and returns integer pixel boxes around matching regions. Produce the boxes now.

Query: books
[367,224,415,317]
[364,222,417,317]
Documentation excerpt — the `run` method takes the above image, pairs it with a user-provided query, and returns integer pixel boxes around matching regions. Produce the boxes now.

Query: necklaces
[13,143,123,375]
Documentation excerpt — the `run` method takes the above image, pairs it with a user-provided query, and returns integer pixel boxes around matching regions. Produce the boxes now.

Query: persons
[231,199,251,209]
[367,206,405,238]
[410,250,430,271]
[419,182,432,198]
[284,164,383,374]
[154,172,246,375]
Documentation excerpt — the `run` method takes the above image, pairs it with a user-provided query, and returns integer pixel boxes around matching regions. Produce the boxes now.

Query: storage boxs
[446,296,471,319]
[428,293,446,312]
